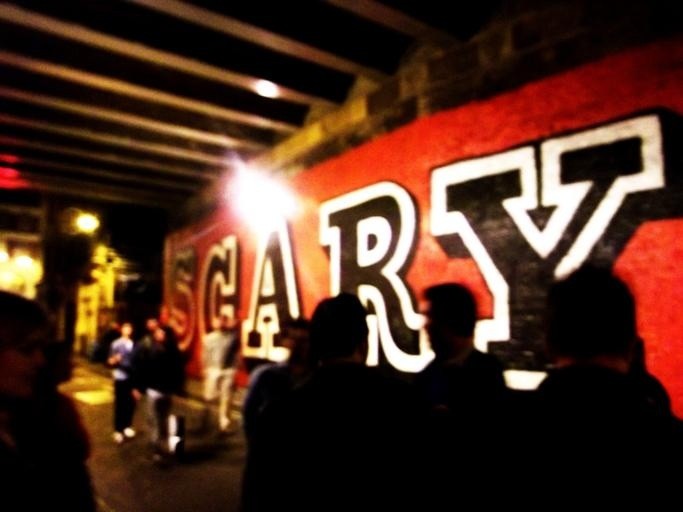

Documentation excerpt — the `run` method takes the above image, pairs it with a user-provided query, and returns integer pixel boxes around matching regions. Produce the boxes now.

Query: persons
[240,263,683,512]
[107,315,239,449]
[1,291,96,512]
